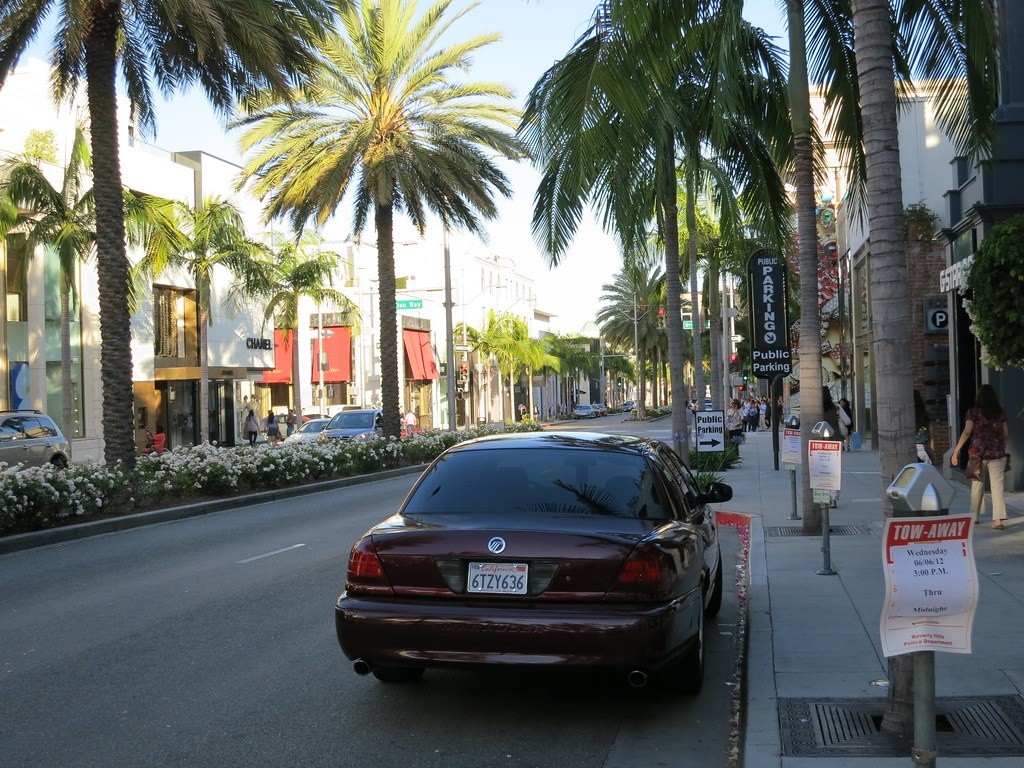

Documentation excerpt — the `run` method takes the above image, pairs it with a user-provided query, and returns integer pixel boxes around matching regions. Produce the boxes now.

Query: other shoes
[763,426,768,431]
[760,429,762,431]
[992,524,1004,530]
[974,520,980,524]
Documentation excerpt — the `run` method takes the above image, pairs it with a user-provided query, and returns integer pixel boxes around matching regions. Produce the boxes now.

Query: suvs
[0,409,72,472]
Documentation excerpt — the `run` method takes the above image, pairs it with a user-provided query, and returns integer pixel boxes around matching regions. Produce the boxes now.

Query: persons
[141,425,166,454]
[952,384,1008,529]
[839,398,854,452]
[518,402,527,419]
[822,386,842,441]
[286,409,293,436]
[685,401,694,454]
[914,389,932,465]
[245,409,259,445]
[535,405,539,421]
[399,406,416,434]
[734,396,783,432]
[264,410,278,446]
[558,402,576,414]
[725,401,744,453]
[240,394,264,435]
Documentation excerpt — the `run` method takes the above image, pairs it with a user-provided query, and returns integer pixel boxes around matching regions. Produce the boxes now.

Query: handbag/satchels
[966,459,981,480]
[838,418,849,436]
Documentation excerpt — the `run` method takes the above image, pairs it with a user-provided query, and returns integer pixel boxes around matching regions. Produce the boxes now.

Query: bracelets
[146,433,149,434]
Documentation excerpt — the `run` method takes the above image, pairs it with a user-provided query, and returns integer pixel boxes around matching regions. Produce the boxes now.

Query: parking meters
[783,415,801,519]
[886,462,957,767]
[810,420,837,576]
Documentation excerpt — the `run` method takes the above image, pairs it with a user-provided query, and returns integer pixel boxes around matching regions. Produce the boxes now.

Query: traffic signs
[695,410,724,453]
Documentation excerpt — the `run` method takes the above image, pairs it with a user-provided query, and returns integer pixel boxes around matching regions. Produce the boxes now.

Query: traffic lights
[738,386,746,392]
[656,306,668,330]
[459,361,470,382]
[730,353,738,373]
[742,368,748,382]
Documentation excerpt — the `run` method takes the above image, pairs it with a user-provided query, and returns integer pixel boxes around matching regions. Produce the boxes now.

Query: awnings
[403,331,440,380]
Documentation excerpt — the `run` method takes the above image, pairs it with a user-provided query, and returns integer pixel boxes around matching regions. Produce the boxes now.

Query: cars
[320,409,384,440]
[574,398,713,418]
[336,431,732,695]
[284,418,330,445]
